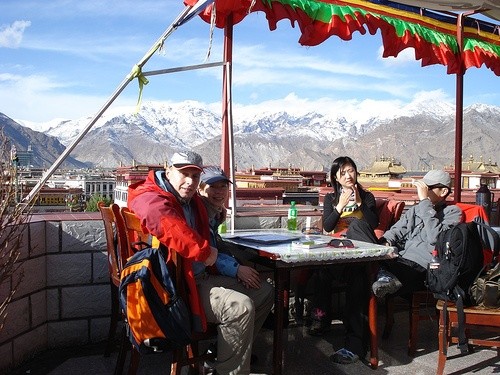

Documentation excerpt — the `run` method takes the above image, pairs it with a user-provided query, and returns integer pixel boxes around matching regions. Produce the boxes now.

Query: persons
[196,165,263,290]
[127,150,275,375]
[330,169,464,364]
[306,156,379,336]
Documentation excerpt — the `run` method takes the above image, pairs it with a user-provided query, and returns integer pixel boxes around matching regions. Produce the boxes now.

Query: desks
[216,225,399,375]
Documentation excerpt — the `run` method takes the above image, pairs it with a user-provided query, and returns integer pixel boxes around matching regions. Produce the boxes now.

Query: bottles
[287,201,297,230]
[218,203,226,233]
[430,250,440,269]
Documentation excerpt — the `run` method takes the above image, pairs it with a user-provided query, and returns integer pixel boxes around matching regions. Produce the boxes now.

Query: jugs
[476,183,494,226]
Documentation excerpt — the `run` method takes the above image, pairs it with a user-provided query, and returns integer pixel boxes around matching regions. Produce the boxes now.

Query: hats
[169,151,206,175]
[199,167,233,184]
[415,170,452,188]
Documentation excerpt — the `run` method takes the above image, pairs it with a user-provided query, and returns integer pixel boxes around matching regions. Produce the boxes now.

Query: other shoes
[331,348,359,364]
[371,270,403,297]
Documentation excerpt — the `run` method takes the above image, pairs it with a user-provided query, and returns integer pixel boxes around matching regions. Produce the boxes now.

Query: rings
[248,278,251,282]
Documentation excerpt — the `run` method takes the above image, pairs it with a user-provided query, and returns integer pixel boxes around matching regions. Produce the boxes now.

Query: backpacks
[424,221,498,305]
[118,236,194,352]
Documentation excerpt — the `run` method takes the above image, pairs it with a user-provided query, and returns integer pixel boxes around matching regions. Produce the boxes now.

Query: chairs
[370,196,500,375]
[95,200,208,375]
